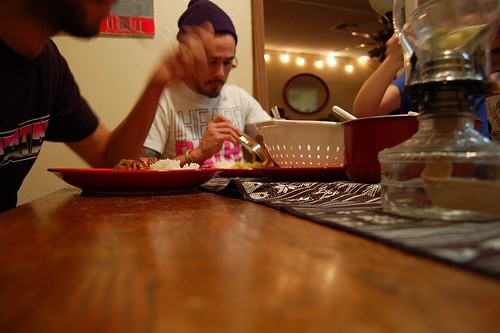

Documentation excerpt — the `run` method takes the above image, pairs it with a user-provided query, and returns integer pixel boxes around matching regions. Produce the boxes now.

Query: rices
[149,157,200,170]
[212,160,237,169]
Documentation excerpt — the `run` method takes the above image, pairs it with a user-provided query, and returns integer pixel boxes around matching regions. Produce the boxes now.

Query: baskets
[257,120,344,168]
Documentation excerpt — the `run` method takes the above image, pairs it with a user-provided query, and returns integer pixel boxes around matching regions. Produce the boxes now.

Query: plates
[46,167,223,195]
[203,166,344,183]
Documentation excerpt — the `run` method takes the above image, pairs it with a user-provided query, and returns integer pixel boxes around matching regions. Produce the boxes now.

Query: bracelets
[185,149,195,163]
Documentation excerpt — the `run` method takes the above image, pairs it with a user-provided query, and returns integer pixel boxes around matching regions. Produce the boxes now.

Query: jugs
[377,0,500,221]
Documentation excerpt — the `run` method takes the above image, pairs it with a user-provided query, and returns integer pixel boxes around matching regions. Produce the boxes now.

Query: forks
[238,135,268,162]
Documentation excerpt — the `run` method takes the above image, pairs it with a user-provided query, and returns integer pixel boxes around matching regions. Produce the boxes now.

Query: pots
[339,113,484,183]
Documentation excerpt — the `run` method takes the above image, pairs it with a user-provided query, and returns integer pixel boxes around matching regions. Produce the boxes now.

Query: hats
[177,0,238,40]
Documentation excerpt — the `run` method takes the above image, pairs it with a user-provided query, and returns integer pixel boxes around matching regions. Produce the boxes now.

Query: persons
[143,0,273,169]
[0,0,216,211]
[352,32,489,139]
